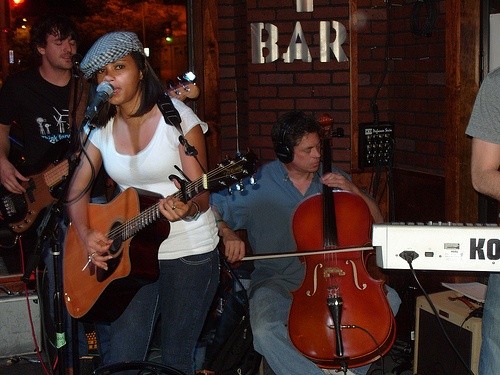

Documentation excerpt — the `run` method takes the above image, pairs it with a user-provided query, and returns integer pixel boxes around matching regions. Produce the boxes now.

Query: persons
[68,31,221,375]
[466,68,500,375]
[1,13,115,365]
[209,111,402,375]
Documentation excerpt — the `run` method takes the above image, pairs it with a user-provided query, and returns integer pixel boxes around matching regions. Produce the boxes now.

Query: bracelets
[215,219,225,224]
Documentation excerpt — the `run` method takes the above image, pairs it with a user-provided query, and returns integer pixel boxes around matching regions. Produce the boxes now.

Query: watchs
[182,200,201,223]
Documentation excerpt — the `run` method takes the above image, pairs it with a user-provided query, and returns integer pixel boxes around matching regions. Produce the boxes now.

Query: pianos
[371,220,500,272]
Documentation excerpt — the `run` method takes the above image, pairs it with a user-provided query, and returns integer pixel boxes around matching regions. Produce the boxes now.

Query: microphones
[79,81,114,130]
[71,54,82,80]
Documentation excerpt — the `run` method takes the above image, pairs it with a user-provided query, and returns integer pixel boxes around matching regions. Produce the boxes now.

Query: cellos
[287,115,395,369]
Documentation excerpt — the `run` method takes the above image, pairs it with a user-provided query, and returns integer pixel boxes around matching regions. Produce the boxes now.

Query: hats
[80,31,143,79]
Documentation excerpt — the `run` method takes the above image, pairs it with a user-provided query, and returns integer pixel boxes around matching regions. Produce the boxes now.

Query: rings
[91,252,98,258]
[172,206,176,210]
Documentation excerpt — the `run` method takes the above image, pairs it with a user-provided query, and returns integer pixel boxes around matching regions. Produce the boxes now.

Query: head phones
[275,112,301,163]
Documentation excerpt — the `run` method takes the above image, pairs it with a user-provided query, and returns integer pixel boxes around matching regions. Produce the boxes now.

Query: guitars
[0,76,200,235]
[62,148,258,325]
